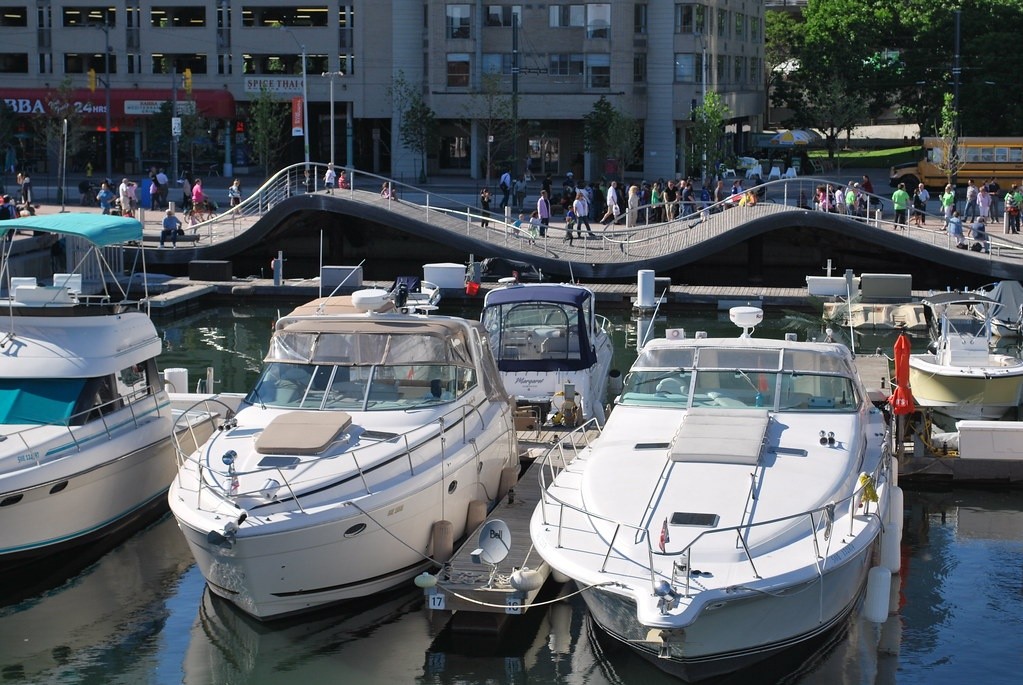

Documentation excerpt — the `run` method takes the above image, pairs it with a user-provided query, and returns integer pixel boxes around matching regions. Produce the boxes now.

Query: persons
[338,171,350,189]
[283,180,291,198]
[481,172,768,246]
[425,379,452,402]
[302,170,315,193]
[524,154,537,183]
[229,178,241,219]
[2,194,15,241]
[80,178,138,216]
[945,211,965,248]
[159,209,182,248]
[815,175,873,219]
[149,168,169,211]
[892,177,1023,234]
[180,169,206,222]
[967,217,989,254]
[381,182,399,201]
[21,172,31,204]
[323,163,336,195]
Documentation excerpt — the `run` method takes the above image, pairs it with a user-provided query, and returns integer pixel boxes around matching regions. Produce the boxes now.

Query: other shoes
[158,244,165,248]
[569,245,575,247]
[578,236,584,239]
[917,225,922,228]
[961,219,966,223]
[614,222,621,225]
[939,228,946,231]
[1012,231,1019,234]
[600,219,606,225]
[688,225,693,229]
[173,245,177,249]
[914,223,918,225]
[589,233,595,237]
[969,220,974,223]
[562,238,566,243]
[1016,227,1020,231]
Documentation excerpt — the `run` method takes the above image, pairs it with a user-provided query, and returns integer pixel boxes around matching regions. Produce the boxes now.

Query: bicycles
[758,193,776,203]
[184,198,219,224]
[869,197,884,212]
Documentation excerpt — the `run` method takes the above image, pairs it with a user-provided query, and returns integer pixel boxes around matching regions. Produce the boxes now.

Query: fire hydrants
[85,163,94,177]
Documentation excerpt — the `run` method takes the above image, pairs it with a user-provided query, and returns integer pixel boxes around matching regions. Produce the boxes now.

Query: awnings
[0,87,236,120]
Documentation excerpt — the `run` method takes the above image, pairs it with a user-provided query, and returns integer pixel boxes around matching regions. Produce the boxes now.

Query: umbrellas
[888,329,915,442]
[769,129,817,145]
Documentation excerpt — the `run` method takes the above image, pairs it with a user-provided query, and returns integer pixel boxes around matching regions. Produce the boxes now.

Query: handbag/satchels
[499,175,509,192]
[613,204,621,216]
[177,229,184,236]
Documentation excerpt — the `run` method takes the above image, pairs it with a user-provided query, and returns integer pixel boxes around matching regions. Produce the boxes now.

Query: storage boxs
[189,260,233,281]
[321,265,363,286]
[422,262,467,287]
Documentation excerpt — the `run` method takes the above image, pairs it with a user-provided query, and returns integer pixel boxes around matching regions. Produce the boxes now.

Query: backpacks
[0,204,12,220]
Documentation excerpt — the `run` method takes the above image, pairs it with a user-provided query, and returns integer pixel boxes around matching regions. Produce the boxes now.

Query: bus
[889,137,1023,196]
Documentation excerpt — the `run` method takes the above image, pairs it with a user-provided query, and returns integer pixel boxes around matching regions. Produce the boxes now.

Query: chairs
[719,163,736,178]
[208,164,220,177]
[746,164,762,179]
[10,277,37,301]
[781,168,797,179]
[768,167,780,180]
[53,274,82,301]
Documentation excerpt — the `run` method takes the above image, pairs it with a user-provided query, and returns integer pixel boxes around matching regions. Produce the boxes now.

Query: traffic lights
[183,69,191,95]
[87,69,95,93]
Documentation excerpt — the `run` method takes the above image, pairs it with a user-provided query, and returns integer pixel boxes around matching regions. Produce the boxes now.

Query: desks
[738,158,759,175]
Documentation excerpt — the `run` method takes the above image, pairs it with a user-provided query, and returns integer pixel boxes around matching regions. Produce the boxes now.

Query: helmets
[566,172,574,177]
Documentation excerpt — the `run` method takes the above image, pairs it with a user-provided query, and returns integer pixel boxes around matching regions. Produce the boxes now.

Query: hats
[3,195,12,200]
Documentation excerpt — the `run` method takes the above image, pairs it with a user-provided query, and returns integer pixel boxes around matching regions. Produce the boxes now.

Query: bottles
[755,392,764,407]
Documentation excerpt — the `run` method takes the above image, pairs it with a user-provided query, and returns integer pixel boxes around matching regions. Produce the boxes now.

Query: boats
[0,212,219,582]
[529,283,899,682]
[909,279,1023,421]
[168,230,613,622]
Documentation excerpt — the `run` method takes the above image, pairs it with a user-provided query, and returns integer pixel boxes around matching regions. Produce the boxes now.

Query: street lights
[694,31,707,189]
[322,72,343,163]
